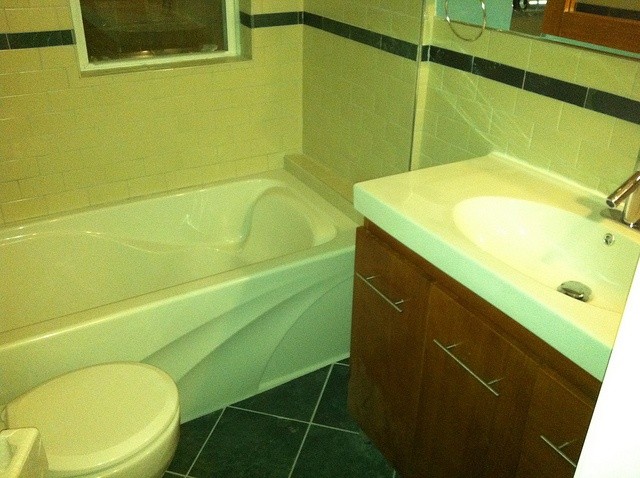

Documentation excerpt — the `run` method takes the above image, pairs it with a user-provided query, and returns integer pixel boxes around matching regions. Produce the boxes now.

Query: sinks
[452,195,639,323]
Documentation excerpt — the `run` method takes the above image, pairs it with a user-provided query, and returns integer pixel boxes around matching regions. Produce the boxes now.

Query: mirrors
[539,0,640,53]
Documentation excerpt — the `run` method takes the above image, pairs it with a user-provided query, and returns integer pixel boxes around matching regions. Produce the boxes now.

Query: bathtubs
[0,169,355,424]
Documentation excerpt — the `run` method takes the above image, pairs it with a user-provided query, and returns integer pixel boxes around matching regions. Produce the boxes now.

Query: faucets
[606,169,639,230]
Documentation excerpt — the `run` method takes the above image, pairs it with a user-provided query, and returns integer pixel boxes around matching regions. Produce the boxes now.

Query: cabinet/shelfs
[347,217,542,478]
[517,340,602,478]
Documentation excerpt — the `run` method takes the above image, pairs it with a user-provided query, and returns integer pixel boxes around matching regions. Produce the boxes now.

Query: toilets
[0,362,182,478]
